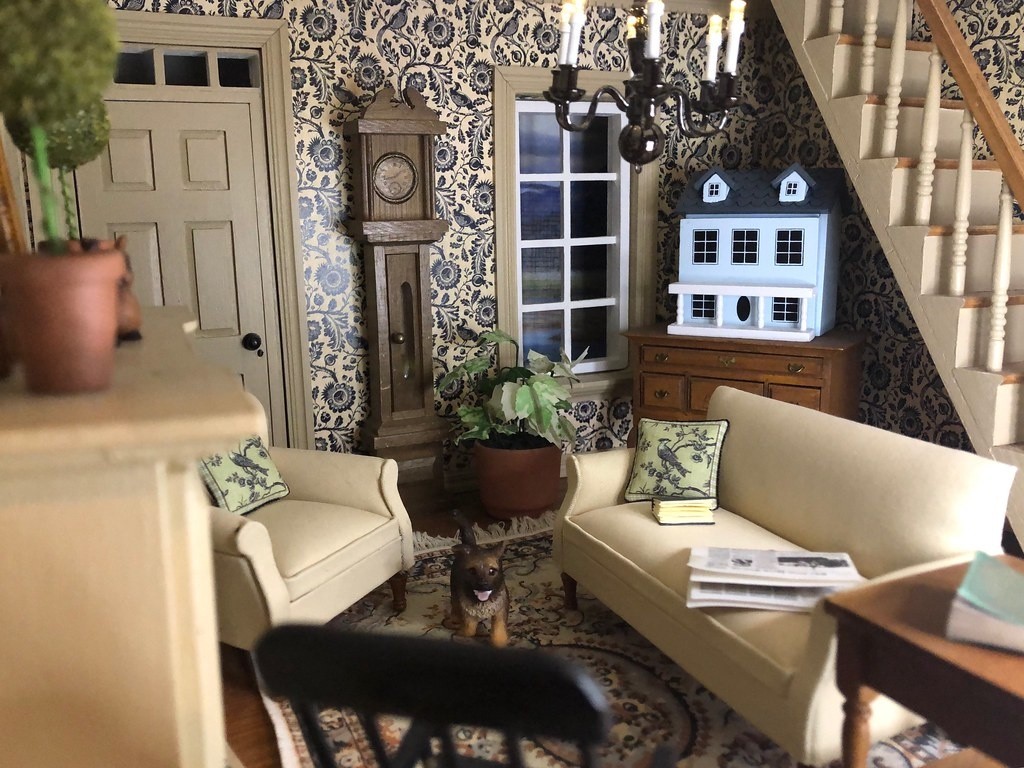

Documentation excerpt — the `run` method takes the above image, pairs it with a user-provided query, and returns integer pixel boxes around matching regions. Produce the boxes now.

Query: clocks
[341,87,451,486]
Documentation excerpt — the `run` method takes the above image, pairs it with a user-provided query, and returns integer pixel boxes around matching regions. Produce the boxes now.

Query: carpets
[249,508,972,768]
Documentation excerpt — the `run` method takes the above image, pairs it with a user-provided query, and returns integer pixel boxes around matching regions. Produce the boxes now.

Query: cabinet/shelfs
[619,323,864,443]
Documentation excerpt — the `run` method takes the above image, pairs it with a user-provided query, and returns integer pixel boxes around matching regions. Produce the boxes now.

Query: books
[686,546,858,612]
[649,496,718,525]
[945,550,1024,653]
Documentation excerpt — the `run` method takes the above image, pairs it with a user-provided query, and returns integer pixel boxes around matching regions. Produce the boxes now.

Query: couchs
[550,386,1017,768]
[201,389,415,662]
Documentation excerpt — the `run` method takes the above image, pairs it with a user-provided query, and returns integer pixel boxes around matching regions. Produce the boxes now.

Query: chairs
[256,624,609,768]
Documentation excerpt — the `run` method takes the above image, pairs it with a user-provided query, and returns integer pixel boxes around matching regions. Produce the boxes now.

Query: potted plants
[0,0,142,399]
[436,330,590,522]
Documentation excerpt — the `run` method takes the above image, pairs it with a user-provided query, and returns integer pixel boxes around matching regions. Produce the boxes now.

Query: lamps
[544,0,747,167]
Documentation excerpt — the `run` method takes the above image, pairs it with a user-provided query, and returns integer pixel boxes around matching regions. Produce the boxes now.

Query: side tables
[823,554,1024,768]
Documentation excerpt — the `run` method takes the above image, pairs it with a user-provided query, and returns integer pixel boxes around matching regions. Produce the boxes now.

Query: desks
[0,306,270,768]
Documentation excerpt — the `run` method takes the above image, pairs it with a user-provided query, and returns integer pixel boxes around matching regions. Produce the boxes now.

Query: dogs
[450,526,509,648]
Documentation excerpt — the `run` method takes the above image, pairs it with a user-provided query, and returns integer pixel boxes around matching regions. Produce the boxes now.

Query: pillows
[199,433,290,516]
[623,417,730,510]
